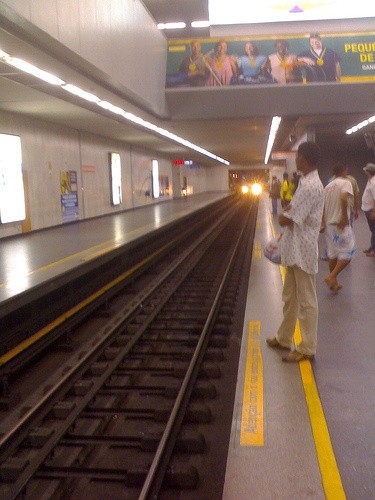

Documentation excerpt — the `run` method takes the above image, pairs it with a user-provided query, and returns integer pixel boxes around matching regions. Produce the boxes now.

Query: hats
[363,163,375,172]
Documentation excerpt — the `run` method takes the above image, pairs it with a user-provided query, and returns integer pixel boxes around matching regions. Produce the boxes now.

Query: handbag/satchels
[264,239,283,264]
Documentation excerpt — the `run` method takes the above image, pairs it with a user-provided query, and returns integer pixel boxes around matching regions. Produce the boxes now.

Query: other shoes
[281,350,315,363]
[364,247,375,256]
[266,338,291,350]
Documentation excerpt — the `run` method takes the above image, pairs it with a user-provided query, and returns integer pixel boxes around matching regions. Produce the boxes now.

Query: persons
[270,166,360,291]
[266,142,327,364]
[179,36,342,85]
[360,161,375,257]
